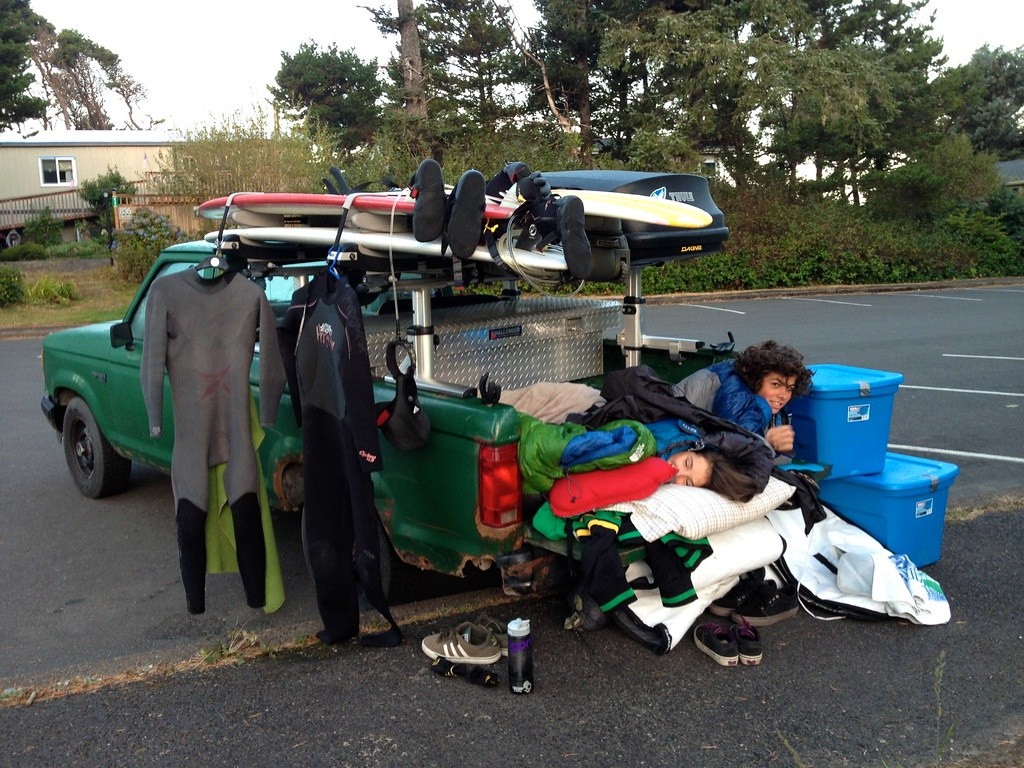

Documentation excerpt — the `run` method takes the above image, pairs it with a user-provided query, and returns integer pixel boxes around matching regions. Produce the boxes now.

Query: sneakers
[469,613,509,656]
[729,617,763,666]
[731,580,798,626]
[709,580,763,616]
[694,623,739,666]
[421,621,500,665]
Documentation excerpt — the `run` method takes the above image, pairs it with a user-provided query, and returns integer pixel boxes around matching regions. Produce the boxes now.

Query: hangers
[195,236,228,271]
[327,243,343,280]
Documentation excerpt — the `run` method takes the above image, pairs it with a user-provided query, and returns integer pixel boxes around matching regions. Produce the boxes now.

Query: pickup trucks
[38,159,750,596]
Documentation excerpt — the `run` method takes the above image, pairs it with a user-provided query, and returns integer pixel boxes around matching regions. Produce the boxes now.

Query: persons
[672,340,817,451]
[644,419,760,503]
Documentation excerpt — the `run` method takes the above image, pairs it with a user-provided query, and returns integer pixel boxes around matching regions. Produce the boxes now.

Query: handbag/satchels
[372,375,430,451]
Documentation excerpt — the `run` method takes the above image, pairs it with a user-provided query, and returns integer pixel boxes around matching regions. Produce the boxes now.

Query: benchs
[376,293,502,315]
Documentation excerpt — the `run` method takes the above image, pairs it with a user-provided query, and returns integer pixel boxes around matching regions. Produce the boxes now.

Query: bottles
[507,620,533,693]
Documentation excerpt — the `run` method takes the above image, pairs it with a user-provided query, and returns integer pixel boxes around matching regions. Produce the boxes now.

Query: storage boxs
[781,364,904,479]
[821,450,960,567]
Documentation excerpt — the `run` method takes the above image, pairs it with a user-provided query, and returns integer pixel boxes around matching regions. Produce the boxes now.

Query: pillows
[616,474,798,541]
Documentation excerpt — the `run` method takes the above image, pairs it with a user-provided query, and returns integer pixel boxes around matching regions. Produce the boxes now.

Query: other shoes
[447,170,486,259]
[555,195,592,279]
[412,159,445,242]
